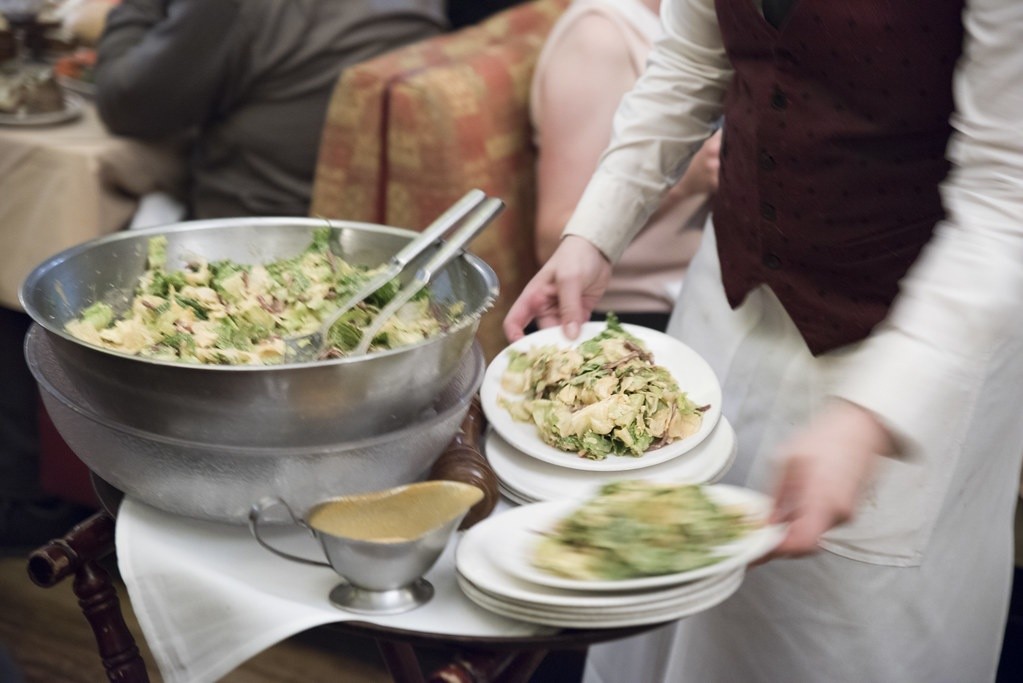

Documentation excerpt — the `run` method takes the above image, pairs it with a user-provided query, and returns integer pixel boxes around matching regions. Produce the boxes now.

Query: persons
[503,0,1023,683]
[51,0,450,221]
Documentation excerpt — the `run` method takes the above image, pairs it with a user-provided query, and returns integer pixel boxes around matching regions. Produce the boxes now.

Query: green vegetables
[74,222,466,364]
[498,310,709,462]
[536,477,746,579]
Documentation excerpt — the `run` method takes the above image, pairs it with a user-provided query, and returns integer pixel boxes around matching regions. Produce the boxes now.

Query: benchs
[305,0,571,362]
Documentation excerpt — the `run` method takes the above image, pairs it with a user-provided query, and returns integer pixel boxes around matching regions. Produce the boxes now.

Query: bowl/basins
[23,321,486,527]
[21,216,502,449]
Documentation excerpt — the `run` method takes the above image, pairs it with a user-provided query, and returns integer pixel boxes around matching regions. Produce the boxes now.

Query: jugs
[250,483,484,614]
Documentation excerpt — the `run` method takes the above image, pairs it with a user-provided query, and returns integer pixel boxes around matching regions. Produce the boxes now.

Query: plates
[0,97,80,125]
[452,323,790,629]
